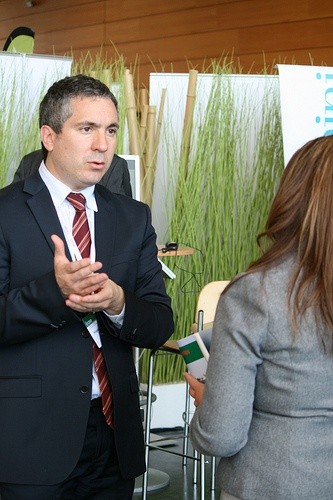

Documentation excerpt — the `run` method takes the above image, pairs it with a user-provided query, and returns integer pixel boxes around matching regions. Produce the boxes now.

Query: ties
[66,192,114,430]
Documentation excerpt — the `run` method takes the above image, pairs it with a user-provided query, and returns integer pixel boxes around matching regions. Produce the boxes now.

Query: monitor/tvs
[119,154,141,201]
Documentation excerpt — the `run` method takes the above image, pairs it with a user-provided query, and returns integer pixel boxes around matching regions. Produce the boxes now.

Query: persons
[160,133,330,500]
[0,74,175,500]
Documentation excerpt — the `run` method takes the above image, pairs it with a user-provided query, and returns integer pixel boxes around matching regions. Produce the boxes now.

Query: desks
[131,245,192,492]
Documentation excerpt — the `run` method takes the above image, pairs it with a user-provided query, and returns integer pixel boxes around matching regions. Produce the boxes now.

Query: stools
[138,280,229,500]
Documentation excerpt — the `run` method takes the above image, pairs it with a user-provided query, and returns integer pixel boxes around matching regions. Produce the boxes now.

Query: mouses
[166,241,178,251]
